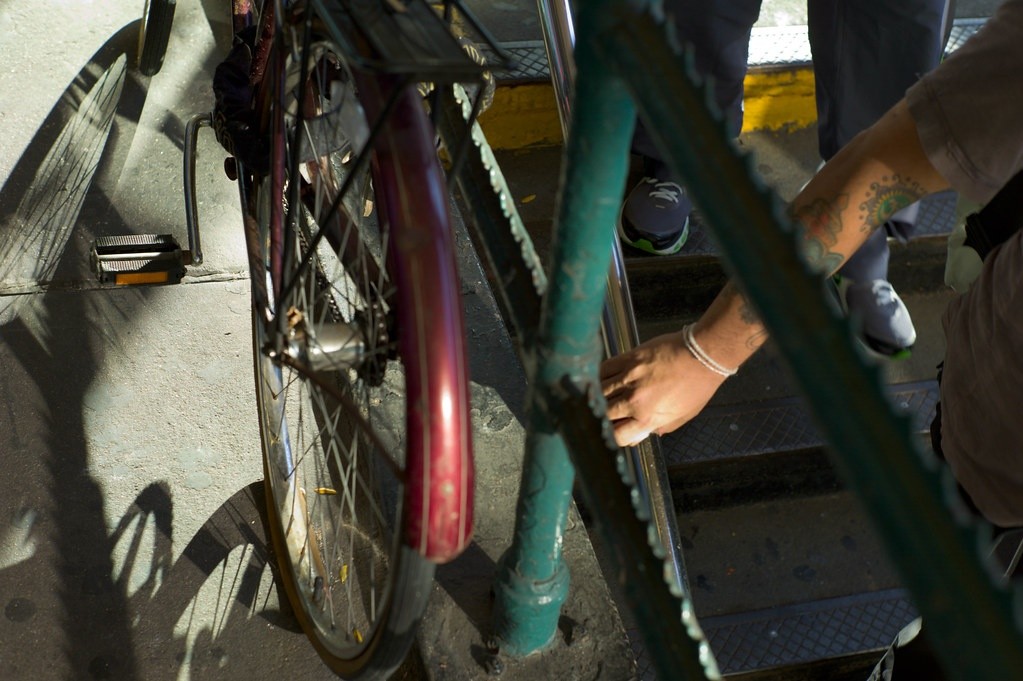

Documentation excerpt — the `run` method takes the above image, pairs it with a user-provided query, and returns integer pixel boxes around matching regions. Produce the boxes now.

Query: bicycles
[90,0,520,681]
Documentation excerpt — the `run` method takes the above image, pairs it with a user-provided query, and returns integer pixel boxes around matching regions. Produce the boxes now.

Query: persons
[589,0,1023,529]
[619,0,958,361]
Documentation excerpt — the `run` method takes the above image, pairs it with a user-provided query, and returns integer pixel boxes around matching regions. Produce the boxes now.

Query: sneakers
[618,170,691,253]
[841,279,918,360]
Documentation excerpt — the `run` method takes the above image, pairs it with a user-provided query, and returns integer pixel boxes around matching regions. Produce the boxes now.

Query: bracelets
[688,320,738,375]
[681,325,730,379]
